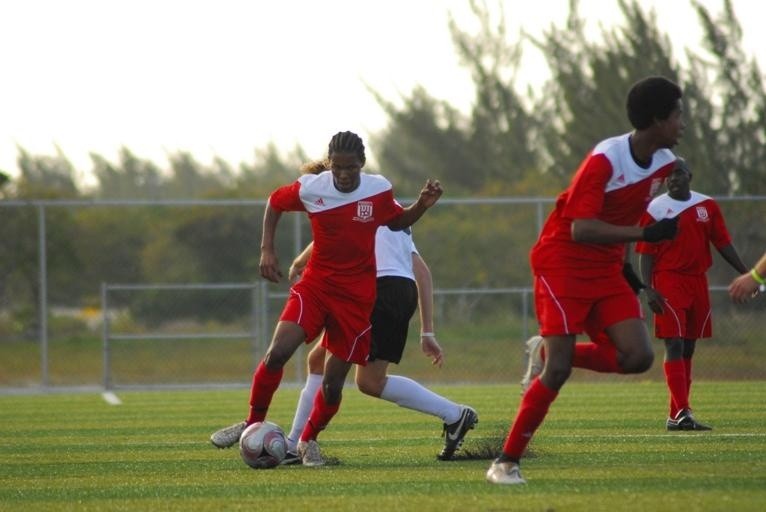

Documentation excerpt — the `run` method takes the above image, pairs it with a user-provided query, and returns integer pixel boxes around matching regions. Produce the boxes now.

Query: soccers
[239,422,286,470]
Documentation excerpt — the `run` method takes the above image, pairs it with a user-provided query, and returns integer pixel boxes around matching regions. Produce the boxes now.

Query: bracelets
[751,267,766,284]
[420,332,434,336]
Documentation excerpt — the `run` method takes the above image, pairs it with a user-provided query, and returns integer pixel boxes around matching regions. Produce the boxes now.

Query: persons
[209,131,443,468]
[280,161,479,466]
[634,156,759,431]
[728,253,766,305]
[485,77,687,486]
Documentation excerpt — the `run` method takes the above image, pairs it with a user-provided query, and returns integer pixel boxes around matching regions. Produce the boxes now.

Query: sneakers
[666,418,679,430]
[678,414,713,432]
[285,436,299,456]
[296,437,325,468]
[437,405,478,460]
[520,334,546,398]
[486,459,528,486]
[210,421,248,450]
